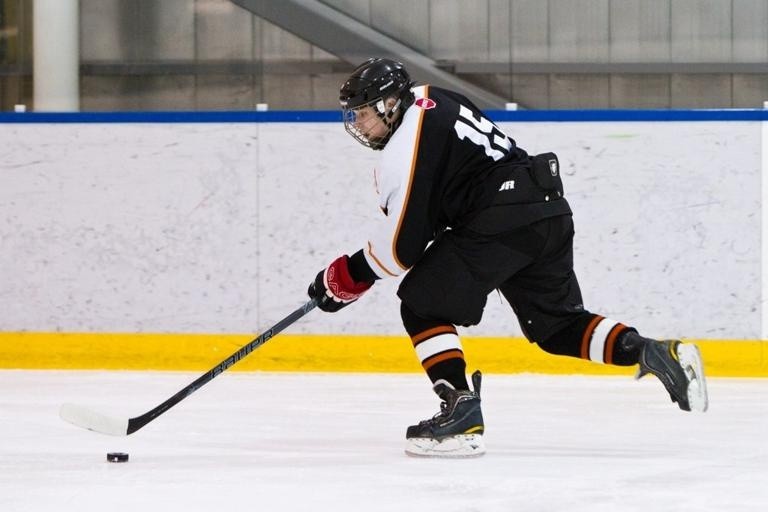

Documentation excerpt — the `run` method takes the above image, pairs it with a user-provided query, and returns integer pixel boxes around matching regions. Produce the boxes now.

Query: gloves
[308,253,373,312]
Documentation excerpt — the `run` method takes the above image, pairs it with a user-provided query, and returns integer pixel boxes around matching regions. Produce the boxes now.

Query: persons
[307,58,709,456]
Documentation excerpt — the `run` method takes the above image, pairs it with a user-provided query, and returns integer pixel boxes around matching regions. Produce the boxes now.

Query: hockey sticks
[60,292,332,436]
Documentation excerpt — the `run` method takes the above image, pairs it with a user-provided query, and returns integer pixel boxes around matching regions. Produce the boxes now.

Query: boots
[406,371,484,438]
[619,331,689,412]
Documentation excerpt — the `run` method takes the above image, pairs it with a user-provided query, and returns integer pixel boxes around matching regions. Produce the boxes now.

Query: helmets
[339,57,415,151]
